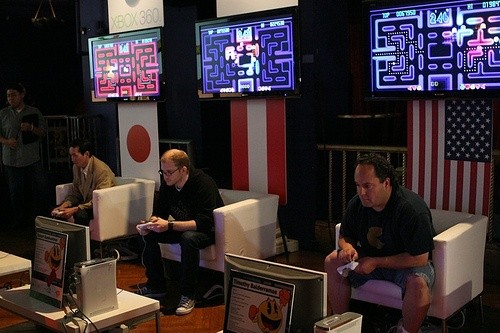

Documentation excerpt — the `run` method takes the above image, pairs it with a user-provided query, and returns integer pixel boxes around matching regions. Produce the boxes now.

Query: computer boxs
[75,257,118,318]
[314,312,363,333]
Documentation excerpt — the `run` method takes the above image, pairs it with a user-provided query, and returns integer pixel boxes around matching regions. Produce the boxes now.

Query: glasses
[158,167,181,176]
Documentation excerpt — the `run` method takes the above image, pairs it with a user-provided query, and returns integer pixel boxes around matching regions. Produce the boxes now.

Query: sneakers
[175,296,196,314]
[135,285,166,296]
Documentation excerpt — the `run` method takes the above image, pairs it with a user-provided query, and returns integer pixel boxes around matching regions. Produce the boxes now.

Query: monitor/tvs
[361,0,500,102]
[29,216,90,309]
[225,253,328,333]
[195,5,304,103]
[88,26,164,104]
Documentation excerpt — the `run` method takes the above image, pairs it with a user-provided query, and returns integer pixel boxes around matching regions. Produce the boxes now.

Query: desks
[0,250,33,292]
[0,284,161,333]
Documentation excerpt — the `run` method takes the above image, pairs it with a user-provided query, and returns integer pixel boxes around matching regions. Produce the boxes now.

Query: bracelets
[30,124,34,131]
[167,220,174,231]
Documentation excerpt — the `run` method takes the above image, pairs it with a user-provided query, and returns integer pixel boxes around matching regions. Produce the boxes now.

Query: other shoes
[397,320,422,333]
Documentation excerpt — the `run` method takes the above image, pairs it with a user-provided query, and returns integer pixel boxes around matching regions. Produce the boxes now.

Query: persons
[133,149,225,314]
[0,84,45,230]
[52,137,119,226]
[325,154,436,333]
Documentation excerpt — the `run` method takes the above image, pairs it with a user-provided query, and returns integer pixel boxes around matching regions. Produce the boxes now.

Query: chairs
[333,208,489,333]
[147,188,280,302]
[55,176,156,263]
